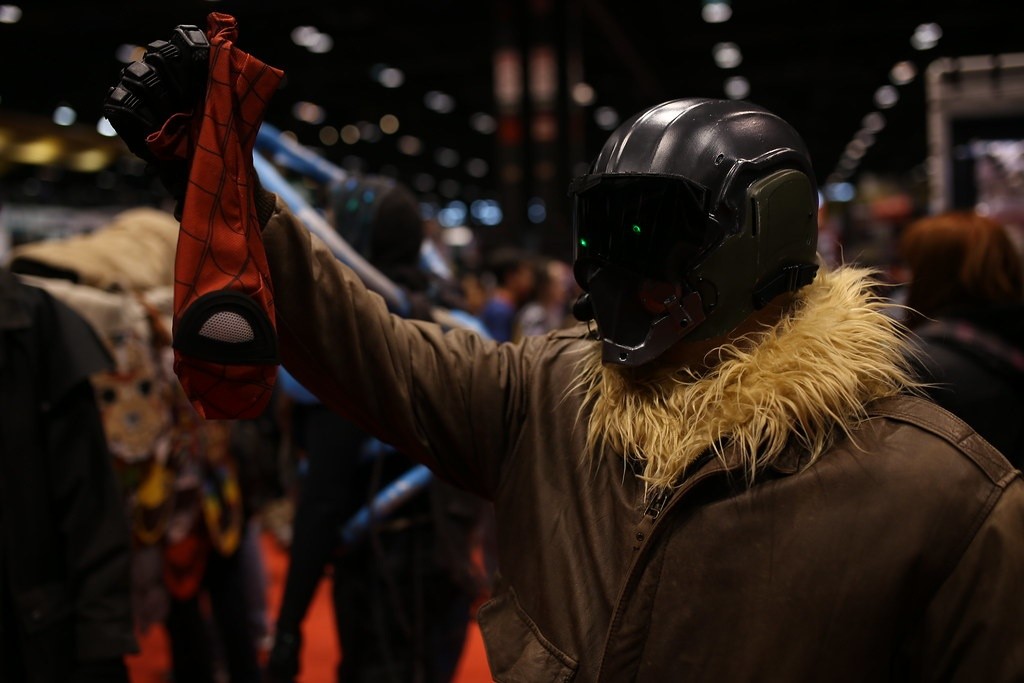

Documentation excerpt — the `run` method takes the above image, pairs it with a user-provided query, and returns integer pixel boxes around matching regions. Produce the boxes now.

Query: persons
[0,172,592,683]
[104,10,1024,683]
[814,152,1024,473]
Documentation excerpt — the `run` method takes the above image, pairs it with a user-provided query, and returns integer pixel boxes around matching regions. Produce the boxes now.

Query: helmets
[569,94,821,370]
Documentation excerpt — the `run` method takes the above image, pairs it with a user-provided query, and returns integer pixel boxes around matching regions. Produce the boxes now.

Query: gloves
[102,22,224,206]
